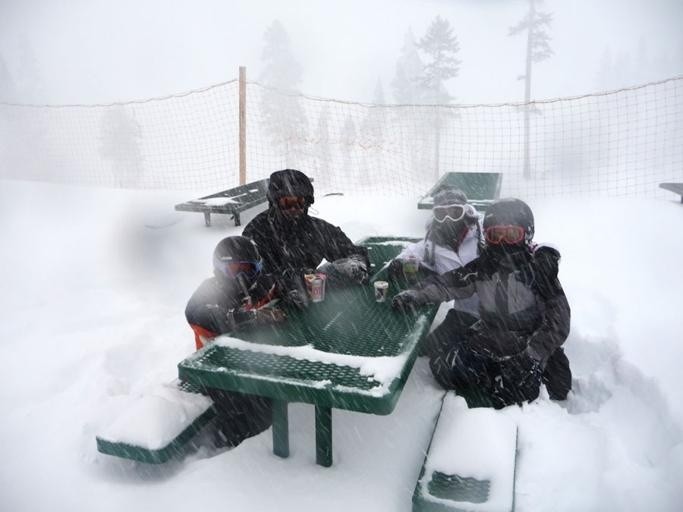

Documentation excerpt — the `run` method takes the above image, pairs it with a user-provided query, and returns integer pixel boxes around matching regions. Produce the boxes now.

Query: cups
[373,280,389,303]
[309,274,327,303]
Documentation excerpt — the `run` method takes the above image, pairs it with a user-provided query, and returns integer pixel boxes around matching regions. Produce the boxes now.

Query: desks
[414,173,505,219]
[175,173,316,228]
[177,232,458,467]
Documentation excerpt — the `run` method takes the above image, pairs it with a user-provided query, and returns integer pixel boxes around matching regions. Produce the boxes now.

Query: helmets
[267,169,314,206]
[212,236,261,286]
[483,198,534,245]
[432,184,468,206]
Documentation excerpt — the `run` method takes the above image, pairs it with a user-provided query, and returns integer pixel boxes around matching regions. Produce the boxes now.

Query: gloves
[255,306,286,324]
[391,289,425,311]
[502,344,543,388]
[286,288,306,311]
[388,259,405,281]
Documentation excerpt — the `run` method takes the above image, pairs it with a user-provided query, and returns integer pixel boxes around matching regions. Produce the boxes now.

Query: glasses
[485,225,523,245]
[219,257,259,280]
[279,200,305,210]
[432,203,465,222]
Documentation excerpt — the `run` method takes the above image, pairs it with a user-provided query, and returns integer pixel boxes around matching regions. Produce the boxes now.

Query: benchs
[410,387,521,512]
[96,343,215,467]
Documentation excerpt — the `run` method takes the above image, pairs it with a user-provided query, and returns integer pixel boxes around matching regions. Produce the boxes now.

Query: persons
[390,197,572,409]
[241,169,371,297]
[370,185,572,400]
[182,234,309,458]
